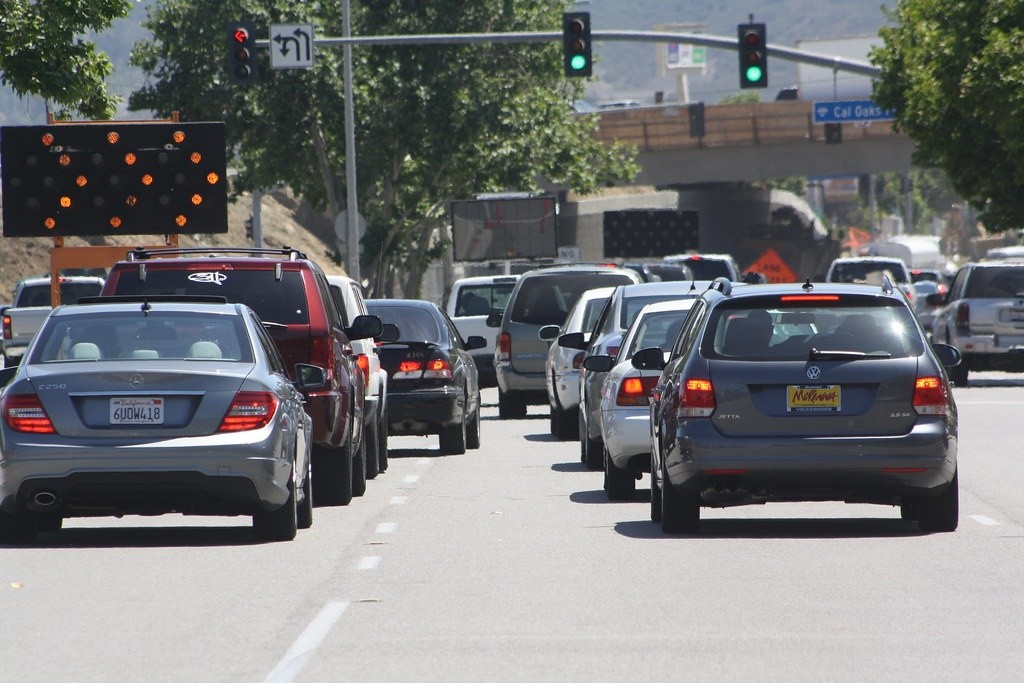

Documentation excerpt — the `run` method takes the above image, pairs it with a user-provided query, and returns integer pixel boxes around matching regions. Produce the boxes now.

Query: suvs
[926,247,1023,390]
[99,245,384,510]
[630,276,962,532]
[320,274,401,480]
[446,273,523,386]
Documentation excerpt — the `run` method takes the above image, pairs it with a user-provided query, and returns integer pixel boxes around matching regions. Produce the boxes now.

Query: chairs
[465,297,490,316]
[69,343,101,358]
[723,319,749,349]
[833,315,905,358]
[187,342,222,359]
[33,293,49,305]
[658,320,683,351]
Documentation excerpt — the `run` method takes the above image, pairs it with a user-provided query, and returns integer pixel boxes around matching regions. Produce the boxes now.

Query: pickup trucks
[0,276,108,370]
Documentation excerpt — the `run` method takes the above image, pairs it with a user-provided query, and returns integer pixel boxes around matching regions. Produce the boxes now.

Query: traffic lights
[562,11,592,78]
[738,23,769,93]
[227,21,259,86]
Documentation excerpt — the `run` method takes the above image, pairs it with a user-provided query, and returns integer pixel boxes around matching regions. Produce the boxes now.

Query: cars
[358,298,488,455]
[909,266,964,330]
[482,253,741,419]
[578,295,821,499]
[1,296,326,542]
[810,256,917,316]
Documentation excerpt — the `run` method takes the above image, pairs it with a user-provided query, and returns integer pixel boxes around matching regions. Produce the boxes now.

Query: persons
[80,324,122,357]
[747,310,775,347]
